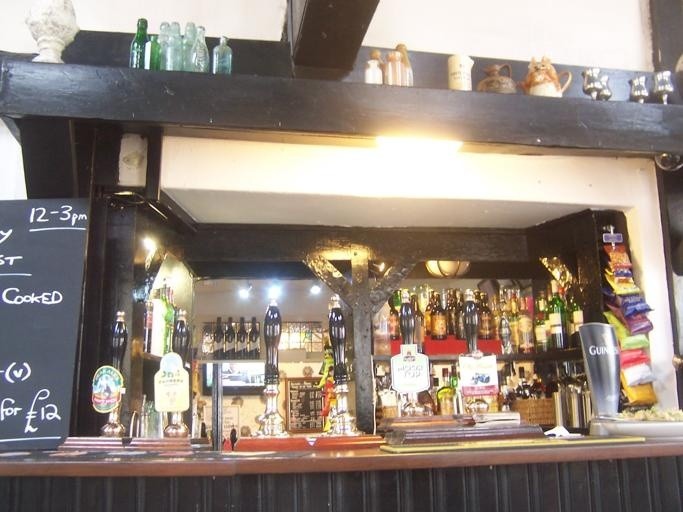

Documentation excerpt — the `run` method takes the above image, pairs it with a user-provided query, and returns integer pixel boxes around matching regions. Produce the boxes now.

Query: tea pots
[476,63,518,94]
[515,56,573,98]
[447,55,474,91]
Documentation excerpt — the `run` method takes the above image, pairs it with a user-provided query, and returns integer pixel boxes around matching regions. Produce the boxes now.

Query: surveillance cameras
[654,151,683,172]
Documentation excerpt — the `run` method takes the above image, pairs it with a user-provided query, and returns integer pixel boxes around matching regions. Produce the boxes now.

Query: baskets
[510,398,556,426]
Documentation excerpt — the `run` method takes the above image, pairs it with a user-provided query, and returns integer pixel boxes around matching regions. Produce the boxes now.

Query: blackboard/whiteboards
[0,197,93,451]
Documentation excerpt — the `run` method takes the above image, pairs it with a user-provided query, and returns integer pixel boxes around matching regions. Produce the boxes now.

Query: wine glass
[581,66,674,105]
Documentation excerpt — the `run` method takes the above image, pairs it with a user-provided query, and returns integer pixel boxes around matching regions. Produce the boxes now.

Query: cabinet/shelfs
[372,346,590,437]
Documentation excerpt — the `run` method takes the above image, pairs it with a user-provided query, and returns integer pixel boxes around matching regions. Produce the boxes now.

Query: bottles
[24,0,80,63]
[389,282,534,354]
[213,317,259,360]
[128,18,232,75]
[535,278,584,352]
[430,365,460,416]
[364,43,414,87]
[512,363,566,399]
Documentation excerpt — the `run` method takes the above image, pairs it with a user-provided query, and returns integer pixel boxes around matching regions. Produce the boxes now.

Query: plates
[600,419,683,442]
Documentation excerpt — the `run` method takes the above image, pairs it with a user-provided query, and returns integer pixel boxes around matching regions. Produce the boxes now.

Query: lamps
[424,260,471,279]
